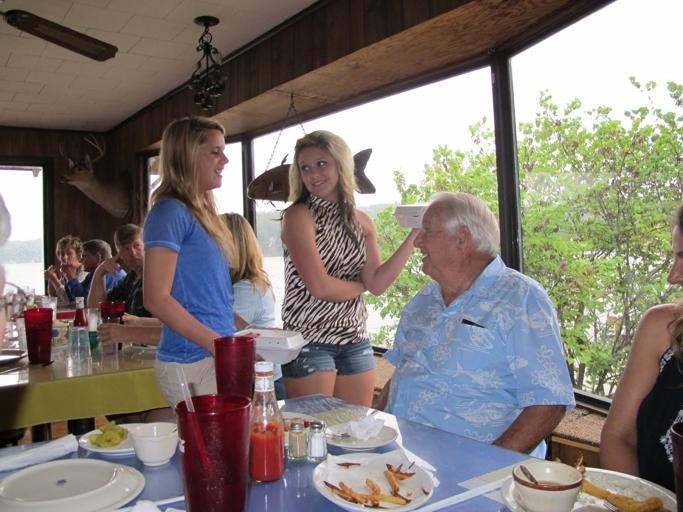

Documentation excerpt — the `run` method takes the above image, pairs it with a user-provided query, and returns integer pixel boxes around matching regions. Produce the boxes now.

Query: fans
[0,0,118,63]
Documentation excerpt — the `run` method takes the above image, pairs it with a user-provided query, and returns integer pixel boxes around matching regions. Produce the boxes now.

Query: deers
[58,134,132,220]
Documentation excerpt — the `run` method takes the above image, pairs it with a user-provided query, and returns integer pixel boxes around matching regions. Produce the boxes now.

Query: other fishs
[246,148,375,202]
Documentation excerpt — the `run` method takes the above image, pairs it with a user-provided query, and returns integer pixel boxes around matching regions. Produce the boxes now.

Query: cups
[3,286,128,365]
[213,334,256,401]
[174,394,250,510]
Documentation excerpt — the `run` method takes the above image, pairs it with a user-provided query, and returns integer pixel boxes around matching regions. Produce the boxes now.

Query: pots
[53,320,69,341]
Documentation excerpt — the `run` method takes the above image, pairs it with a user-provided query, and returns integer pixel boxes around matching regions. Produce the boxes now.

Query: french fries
[323,462,428,508]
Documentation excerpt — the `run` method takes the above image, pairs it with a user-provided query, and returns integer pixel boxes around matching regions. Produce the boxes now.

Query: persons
[0,193,12,352]
[96,212,279,423]
[374,192,577,459]
[142,116,264,413]
[86,223,152,318]
[44,234,89,306]
[280,130,421,407]
[60,238,127,306]
[598,202,683,495]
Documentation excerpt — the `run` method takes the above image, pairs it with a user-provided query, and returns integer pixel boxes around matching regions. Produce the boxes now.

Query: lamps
[186,15,228,111]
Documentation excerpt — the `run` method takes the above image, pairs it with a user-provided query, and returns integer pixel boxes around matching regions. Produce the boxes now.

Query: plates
[500,466,676,511]
[80,421,139,459]
[0,349,27,368]
[276,411,320,445]
[0,456,148,512]
[309,452,434,512]
[325,421,400,452]
[129,342,159,349]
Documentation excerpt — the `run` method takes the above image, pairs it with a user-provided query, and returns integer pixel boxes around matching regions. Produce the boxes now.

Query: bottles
[289,419,327,464]
[250,361,285,482]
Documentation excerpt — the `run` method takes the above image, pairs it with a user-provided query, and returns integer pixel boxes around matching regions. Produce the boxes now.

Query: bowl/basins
[509,461,584,511]
[130,421,178,467]
[136,467,184,500]
